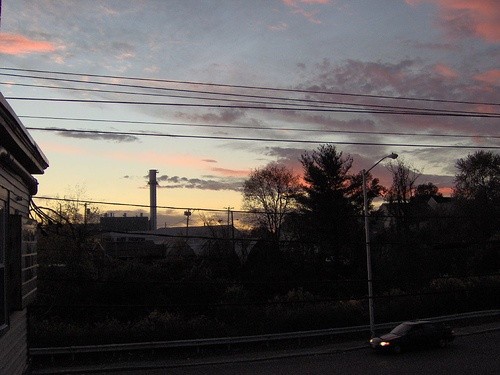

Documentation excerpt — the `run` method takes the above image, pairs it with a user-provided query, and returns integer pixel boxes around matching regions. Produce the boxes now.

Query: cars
[369,319,456,354]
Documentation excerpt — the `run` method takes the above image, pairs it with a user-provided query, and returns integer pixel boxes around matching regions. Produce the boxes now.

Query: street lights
[361,151,399,343]
